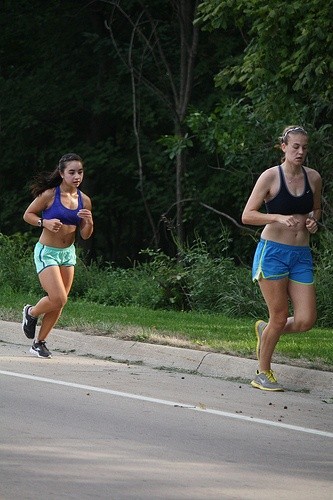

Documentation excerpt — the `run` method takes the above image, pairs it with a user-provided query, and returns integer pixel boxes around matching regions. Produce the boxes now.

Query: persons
[23,153,93,359]
[241,125,322,392]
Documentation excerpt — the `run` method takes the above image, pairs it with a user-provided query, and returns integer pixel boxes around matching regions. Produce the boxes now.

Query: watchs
[37,217,45,228]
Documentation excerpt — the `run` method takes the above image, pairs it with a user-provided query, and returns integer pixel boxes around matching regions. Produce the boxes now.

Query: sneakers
[250,369,284,391]
[28,339,51,359]
[23,304,37,339]
[254,319,270,360]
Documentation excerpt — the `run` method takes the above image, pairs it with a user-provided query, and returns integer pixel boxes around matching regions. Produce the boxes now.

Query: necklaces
[281,164,302,184]
